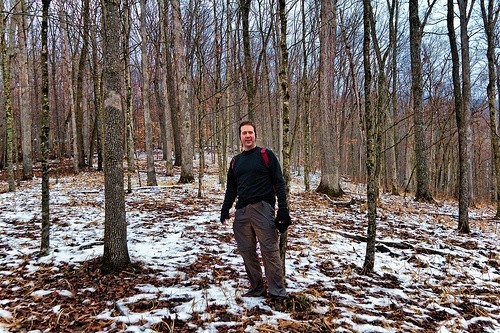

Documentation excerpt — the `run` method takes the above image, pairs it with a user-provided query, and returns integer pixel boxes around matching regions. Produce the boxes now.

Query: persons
[220,122,292,298]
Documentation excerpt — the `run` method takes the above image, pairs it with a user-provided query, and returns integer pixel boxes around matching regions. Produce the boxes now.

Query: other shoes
[270,294,287,303]
[241,285,267,298]
